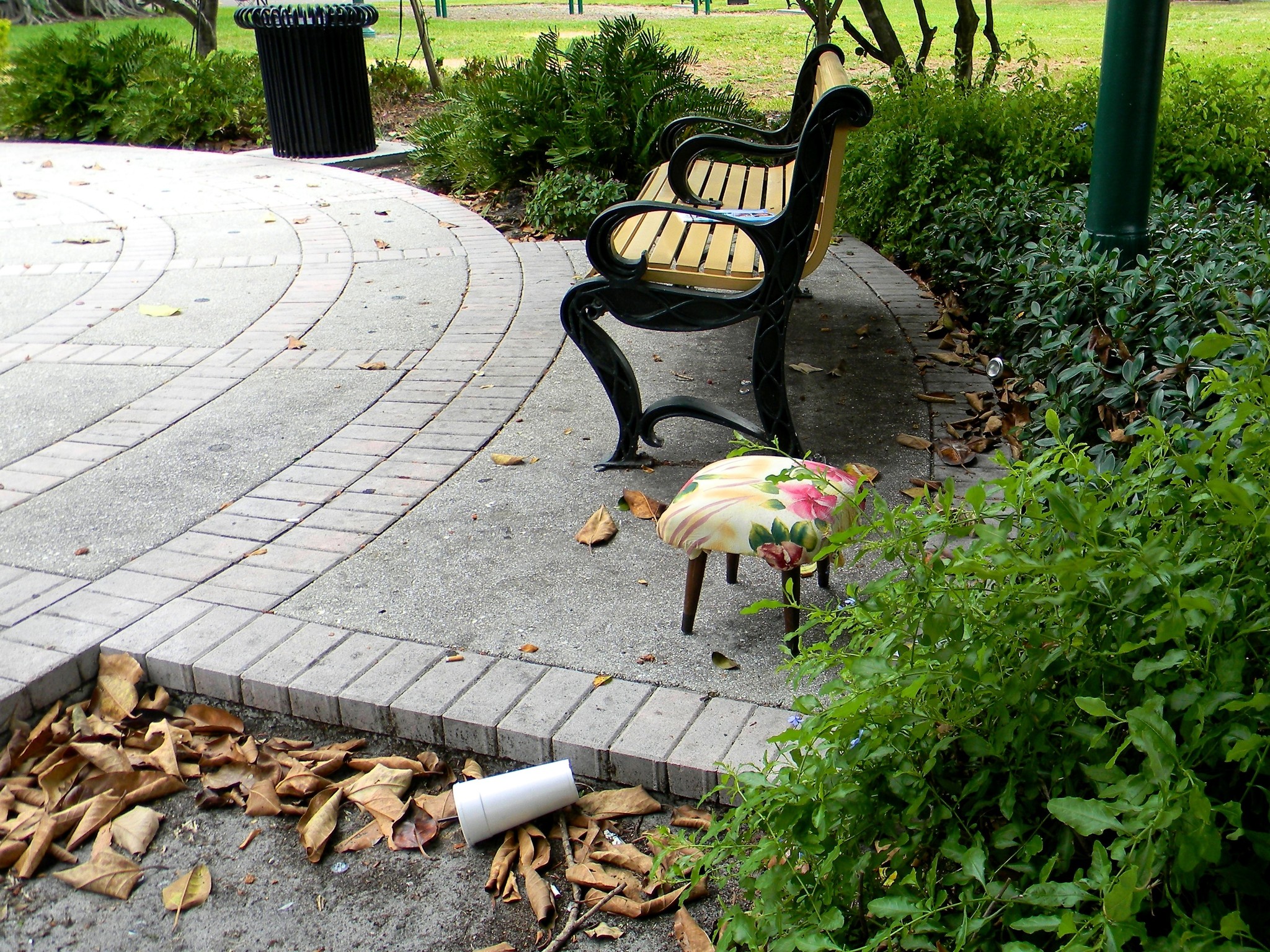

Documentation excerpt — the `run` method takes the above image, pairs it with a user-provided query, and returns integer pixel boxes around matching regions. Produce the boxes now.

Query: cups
[453,759,581,849]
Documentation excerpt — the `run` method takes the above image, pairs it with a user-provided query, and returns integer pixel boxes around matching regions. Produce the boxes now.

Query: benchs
[559,42,874,476]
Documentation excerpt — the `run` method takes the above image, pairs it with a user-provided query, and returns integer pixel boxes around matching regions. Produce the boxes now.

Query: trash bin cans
[235,1,383,161]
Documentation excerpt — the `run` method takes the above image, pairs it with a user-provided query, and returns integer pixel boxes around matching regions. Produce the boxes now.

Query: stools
[655,456,866,660]
